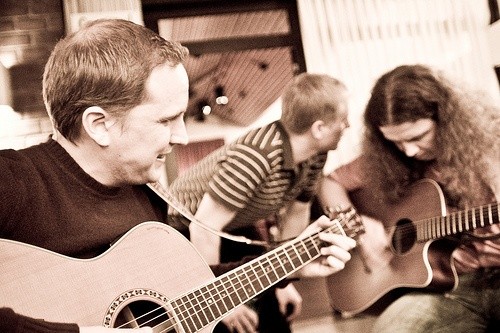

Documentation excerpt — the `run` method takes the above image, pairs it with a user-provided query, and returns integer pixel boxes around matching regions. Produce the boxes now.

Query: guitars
[0,206,367,332]
[325,178,500,315]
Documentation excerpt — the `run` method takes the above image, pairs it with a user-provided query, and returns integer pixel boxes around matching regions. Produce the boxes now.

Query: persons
[0,20,358,333]
[168,72,351,333]
[317,63,500,333]
